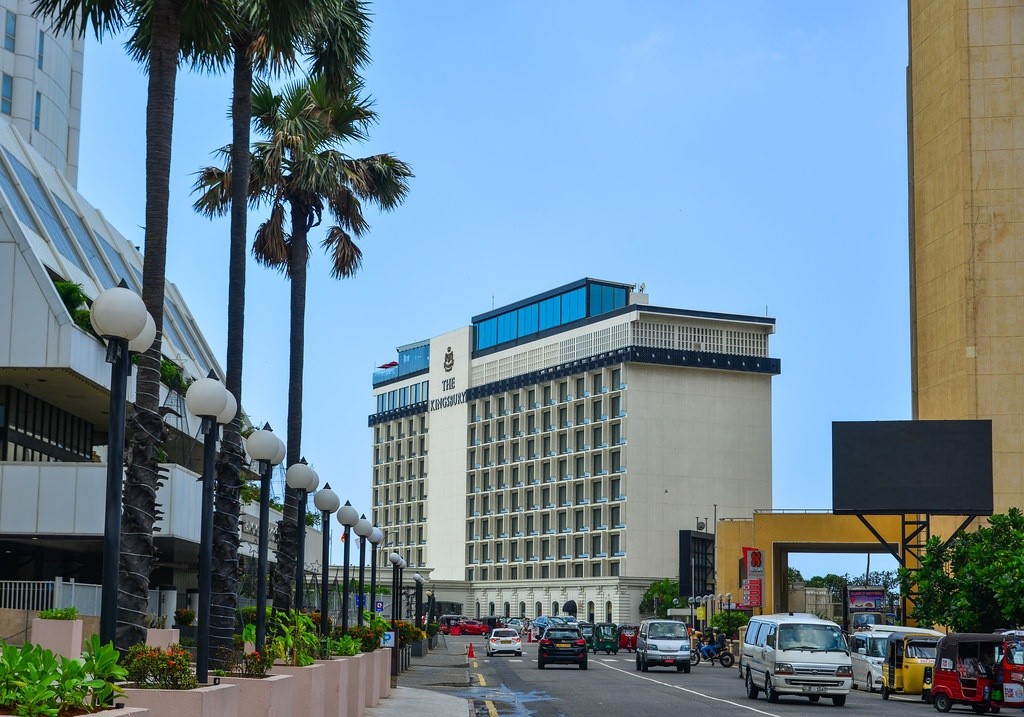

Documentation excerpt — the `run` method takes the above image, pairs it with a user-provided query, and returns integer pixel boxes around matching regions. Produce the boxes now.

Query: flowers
[210,645,279,678]
[117,639,200,689]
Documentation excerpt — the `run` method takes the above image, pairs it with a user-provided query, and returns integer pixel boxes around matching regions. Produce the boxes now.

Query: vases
[109,680,237,717]
[208,671,295,717]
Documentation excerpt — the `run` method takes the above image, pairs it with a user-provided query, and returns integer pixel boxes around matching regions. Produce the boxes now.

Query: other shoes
[705,656,711,662]
[712,653,719,658]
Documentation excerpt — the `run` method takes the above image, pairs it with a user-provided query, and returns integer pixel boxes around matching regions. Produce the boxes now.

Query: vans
[868,624,947,639]
[636,620,694,673]
[849,632,893,693]
[741,611,854,708]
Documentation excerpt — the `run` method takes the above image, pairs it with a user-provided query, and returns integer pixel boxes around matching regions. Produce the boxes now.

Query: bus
[422,601,463,624]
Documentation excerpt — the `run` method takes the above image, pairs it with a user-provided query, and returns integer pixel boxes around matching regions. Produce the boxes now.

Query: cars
[485,629,522,656]
[438,615,579,640]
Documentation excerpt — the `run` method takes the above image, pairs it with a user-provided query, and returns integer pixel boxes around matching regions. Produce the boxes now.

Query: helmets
[713,626,721,633]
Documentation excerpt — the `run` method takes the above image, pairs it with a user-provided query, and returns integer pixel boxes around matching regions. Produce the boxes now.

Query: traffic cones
[467,643,476,658]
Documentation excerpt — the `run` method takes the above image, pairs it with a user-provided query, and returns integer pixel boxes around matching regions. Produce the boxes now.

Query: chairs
[962,657,977,695]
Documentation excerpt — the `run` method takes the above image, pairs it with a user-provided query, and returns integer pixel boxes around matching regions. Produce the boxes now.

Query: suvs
[535,625,588,670]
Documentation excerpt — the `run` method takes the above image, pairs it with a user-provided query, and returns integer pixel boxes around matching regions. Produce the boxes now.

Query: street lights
[334,500,360,637]
[673,593,733,639]
[390,552,407,627]
[314,481,341,656]
[366,521,383,629]
[427,591,439,628]
[185,369,240,682]
[89,279,160,702]
[354,512,374,629]
[413,572,425,630]
[286,455,320,613]
[247,422,286,671]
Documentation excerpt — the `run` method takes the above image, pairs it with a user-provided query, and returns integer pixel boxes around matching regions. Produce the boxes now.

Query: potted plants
[239,606,392,716]
[146,613,179,650]
[0,634,150,717]
[30,606,83,665]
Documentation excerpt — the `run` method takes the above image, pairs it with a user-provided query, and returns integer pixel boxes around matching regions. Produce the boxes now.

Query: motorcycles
[690,636,734,668]
[881,632,941,704]
[578,622,638,655]
[931,632,1024,714]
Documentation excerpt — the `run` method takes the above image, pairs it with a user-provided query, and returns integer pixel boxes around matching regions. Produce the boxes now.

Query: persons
[302,606,308,616]
[523,618,530,635]
[709,627,727,658]
[496,616,502,628]
[315,608,321,613]
[697,627,714,660]
[857,626,862,632]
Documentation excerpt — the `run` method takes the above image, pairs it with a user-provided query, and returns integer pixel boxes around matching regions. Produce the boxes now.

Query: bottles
[984,685,990,700]
